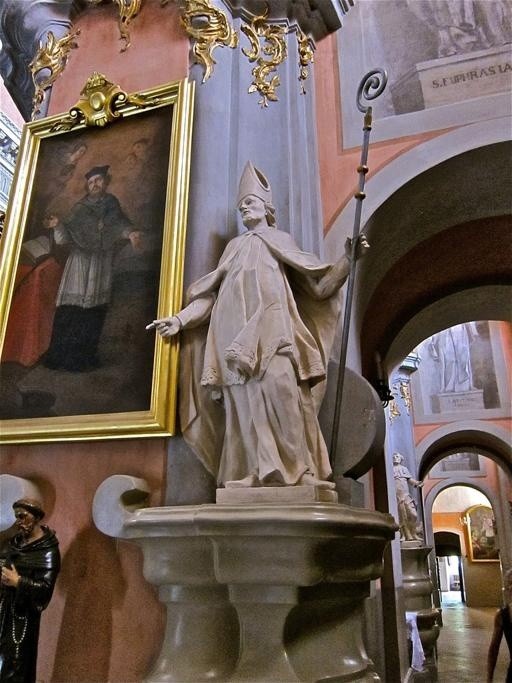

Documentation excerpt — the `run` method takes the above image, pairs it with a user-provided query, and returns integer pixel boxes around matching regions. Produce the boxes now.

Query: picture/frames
[465,504,500,563]
[0,78,196,445]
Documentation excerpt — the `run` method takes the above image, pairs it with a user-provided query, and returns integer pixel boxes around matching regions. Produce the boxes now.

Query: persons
[131,135,168,177]
[145,195,373,493]
[427,321,480,394]
[0,495,60,683]
[124,153,159,204]
[486,567,511,681]
[32,139,88,207]
[43,165,147,375]
[471,515,497,560]
[391,452,429,542]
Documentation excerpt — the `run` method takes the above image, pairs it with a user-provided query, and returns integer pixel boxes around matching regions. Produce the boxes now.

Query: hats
[83,163,112,179]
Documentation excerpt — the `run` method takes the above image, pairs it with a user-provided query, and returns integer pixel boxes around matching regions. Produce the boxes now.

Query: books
[22,232,53,265]
